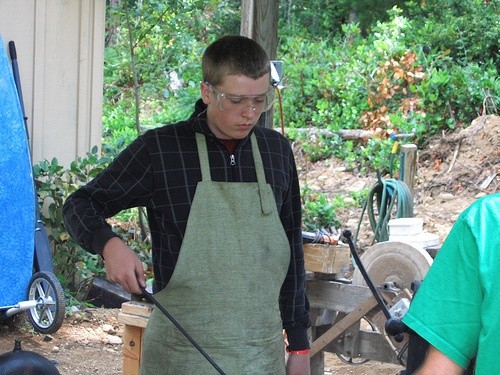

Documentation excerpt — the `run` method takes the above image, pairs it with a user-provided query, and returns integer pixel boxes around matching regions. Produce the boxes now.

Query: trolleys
[0,36,66,334]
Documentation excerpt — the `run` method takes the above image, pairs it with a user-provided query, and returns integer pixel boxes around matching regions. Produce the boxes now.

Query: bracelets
[289,350,310,354]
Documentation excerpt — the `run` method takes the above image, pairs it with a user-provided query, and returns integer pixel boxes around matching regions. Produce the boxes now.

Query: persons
[62,35,312,375]
[402,192,500,375]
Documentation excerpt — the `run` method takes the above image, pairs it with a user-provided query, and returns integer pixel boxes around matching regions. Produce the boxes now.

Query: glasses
[205,81,275,114]
